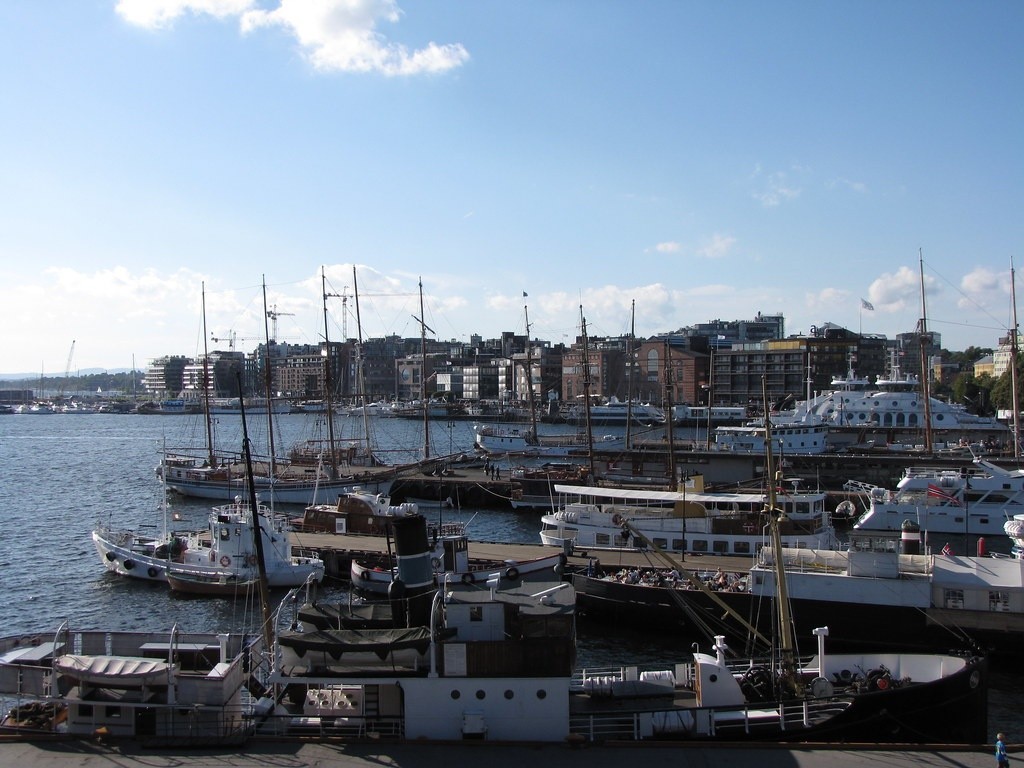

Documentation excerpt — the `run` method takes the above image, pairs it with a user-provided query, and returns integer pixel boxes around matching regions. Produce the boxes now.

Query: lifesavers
[219,554,233,568]
[428,556,443,570]
[504,564,522,582]
[358,568,371,583]
[147,566,159,579]
[460,571,478,587]
[122,557,134,571]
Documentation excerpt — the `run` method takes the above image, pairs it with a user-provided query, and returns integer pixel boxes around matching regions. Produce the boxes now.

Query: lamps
[487,578,500,600]
[489,572,501,593]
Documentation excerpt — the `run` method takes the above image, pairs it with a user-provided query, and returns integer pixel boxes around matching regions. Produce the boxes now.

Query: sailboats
[90,248,1023,615]
[0,368,984,739]
[1,368,470,698]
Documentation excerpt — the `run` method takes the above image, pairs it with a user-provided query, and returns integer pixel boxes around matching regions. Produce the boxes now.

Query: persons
[996,733,1009,768]
[485,463,489,475]
[495,465,501,481]
[613,567,728,590]
[489,463,495,481]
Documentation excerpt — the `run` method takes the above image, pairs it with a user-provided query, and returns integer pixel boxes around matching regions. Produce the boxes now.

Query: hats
[646,572,650,575]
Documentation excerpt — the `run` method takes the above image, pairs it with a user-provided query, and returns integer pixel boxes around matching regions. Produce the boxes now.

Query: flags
[928,483,962,506]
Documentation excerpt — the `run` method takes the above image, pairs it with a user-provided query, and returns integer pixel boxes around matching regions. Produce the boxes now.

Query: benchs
[16,642,68,665]
[139,642,209,668]
